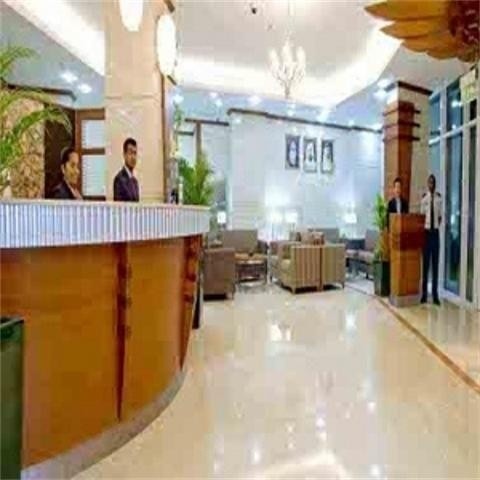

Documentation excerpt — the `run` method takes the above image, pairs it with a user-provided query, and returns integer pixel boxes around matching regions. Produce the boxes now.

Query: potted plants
[177,150,215,329]
[371,195,389,296]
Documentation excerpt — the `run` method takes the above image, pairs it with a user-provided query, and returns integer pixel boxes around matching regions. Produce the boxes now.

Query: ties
[431,193,434,230]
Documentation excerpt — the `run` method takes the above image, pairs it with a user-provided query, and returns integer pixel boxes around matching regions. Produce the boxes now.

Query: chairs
[203,229,378,298]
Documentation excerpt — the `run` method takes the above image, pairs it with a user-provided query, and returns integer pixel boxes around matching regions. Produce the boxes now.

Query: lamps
[266,0,306,98]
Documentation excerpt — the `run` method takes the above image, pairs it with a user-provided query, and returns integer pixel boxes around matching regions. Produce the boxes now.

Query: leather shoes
[421,295,426,302]
[433,294,440,304]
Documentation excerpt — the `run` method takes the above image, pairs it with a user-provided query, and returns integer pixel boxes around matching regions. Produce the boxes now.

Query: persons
[51,146,88,201]
[414,173,444,306]
[110,138,143,201]
[384,175,410,230]
